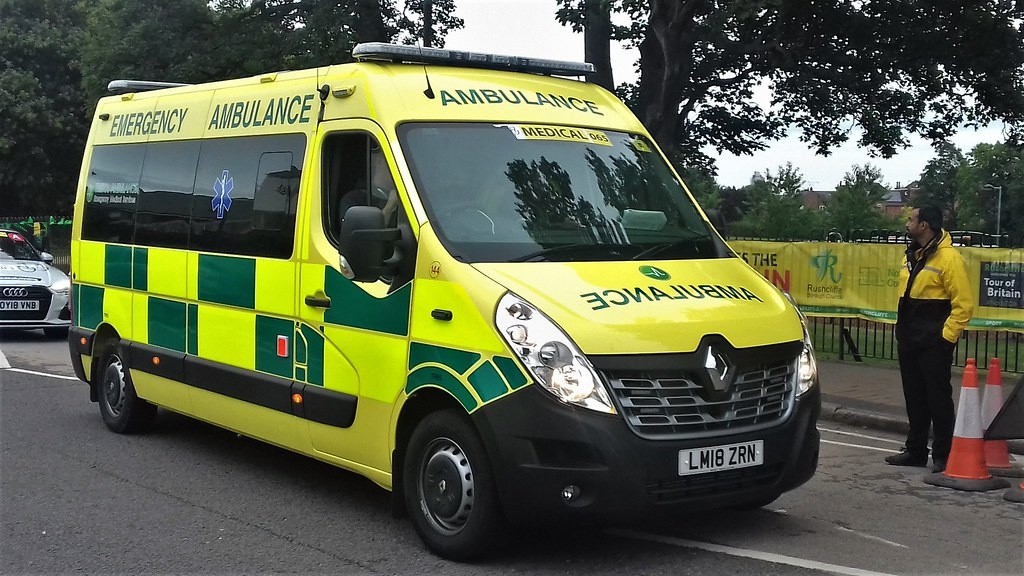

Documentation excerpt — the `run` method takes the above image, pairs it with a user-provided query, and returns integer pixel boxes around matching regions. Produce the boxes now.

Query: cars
[0,229,70,339]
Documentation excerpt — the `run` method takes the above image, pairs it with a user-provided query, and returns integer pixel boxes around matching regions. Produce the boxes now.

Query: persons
[885,202,975,476]
[362,151,397,230]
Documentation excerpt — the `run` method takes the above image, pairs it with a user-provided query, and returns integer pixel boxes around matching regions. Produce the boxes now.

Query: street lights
[982,183,1001,245]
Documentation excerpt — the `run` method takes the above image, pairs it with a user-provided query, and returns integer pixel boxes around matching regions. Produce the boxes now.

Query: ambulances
[65,42,825,558]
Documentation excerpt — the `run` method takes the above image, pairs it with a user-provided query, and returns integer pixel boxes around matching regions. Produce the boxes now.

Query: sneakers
[885,446,927,467]
[931,460,945,472]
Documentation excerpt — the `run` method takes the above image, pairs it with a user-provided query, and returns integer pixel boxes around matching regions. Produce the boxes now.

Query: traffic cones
[980,358,1024,477]
[922,357,1012,493]
